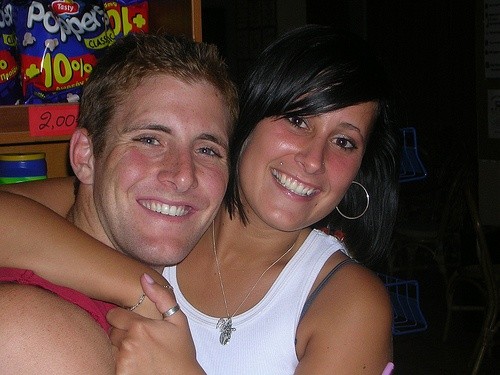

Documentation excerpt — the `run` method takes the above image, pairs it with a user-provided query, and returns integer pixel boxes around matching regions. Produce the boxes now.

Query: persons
[0,29,240,375]
[1,25,398,375]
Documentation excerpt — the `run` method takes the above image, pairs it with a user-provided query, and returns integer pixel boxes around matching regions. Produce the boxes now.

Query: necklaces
[211,219,298,344]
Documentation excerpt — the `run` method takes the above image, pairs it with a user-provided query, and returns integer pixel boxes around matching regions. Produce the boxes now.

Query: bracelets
[126,285,174,312]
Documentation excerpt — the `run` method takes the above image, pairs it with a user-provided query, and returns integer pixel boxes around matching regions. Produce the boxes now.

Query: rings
[161,304,180,318]
